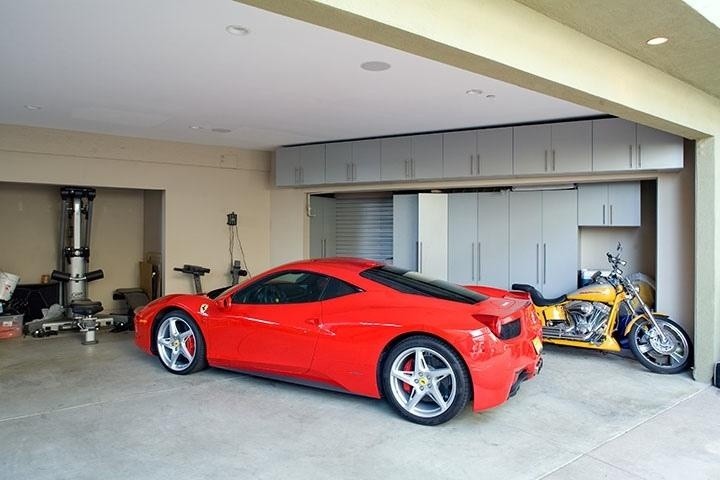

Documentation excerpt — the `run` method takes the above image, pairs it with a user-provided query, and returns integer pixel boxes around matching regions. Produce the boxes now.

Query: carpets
[0,324,710,479]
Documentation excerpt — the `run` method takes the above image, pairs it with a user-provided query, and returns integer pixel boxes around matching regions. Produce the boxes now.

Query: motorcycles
[511,241,694,373]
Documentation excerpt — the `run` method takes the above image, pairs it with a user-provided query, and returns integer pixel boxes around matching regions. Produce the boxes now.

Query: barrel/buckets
[0,267,21,302]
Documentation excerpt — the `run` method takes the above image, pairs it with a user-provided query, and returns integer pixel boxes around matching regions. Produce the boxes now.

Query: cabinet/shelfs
[577,181,642,228]
[275,142,325,188]
[448,193,509,291]
[510,189,581,300]
[443,127,514,186]
[325,139,380,186]
[514,122,592,182]
[593,120,683,176]
[381,133,443,187]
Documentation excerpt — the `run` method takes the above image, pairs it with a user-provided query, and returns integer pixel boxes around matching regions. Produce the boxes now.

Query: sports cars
[132,255,548,426]
